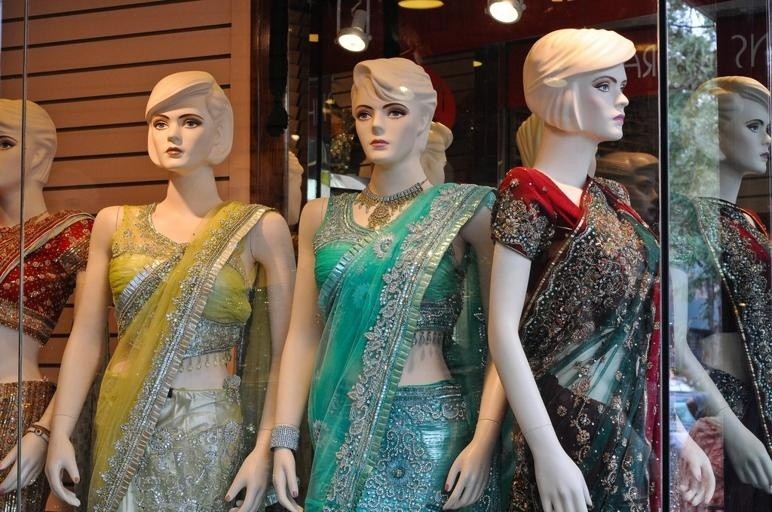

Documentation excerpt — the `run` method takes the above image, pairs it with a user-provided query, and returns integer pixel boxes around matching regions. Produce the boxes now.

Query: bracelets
[28,423,50,443]
[271,425,300,451]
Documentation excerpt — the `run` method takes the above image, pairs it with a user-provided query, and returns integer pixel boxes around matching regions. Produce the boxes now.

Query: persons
[487,27,717,510]
[1,95,95,507]
[45,71,294,512]
[421,121,454,186]
[596,150,658,227]
[514,113,598,178]
[667,74,771,510]
[271,56,508,511]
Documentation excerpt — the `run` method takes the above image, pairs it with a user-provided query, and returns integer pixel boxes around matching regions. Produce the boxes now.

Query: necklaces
[356,178,428,228]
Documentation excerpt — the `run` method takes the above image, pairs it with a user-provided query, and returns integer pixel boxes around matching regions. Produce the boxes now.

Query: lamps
[335,1,372,53]
[484,0,526,24]
